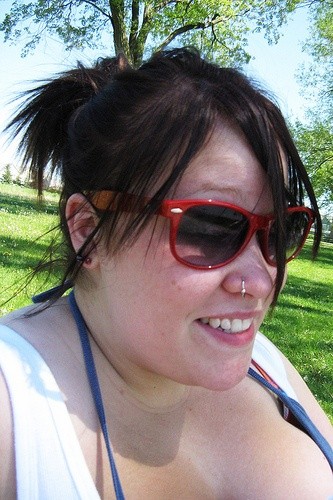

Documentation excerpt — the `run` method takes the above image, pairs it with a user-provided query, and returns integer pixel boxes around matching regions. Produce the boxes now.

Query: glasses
[87,189,314,271]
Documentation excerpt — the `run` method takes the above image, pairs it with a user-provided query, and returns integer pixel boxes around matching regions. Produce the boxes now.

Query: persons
[0,46,333,500]
